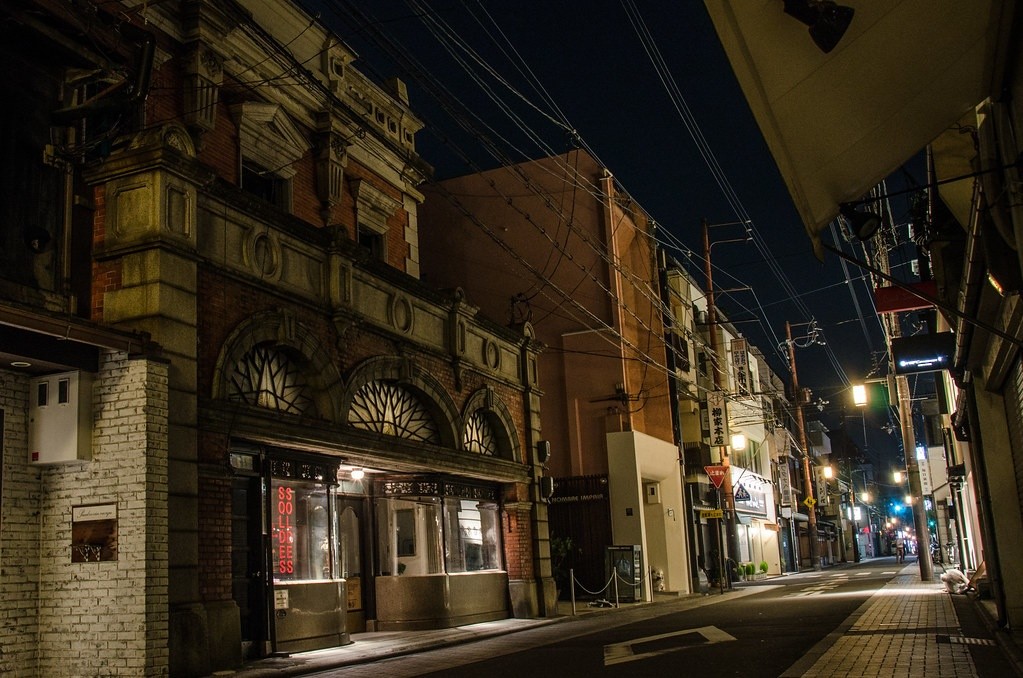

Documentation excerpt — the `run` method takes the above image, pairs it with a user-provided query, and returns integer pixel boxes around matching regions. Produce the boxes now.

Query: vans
[891,541,897,555]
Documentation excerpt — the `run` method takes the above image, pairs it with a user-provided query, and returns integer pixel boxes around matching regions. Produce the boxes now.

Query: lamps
[781,0,855,54]
[351,468,364,479]
[839,174,975,242]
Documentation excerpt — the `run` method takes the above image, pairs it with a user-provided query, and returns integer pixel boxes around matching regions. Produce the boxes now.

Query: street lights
[804,466,832,570]
[850,492,868,563]
[719,430,744,582]
[851,375,933,582]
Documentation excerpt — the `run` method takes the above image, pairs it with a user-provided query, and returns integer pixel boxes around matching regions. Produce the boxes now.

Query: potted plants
[760,561,768,580]
[759,569,765,578]
[746,564,752,581]
[738,567,744,581]
[755,571,761,579]
[750,562,757,580]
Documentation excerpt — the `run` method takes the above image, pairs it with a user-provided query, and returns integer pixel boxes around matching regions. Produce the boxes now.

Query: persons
[899,541,907,560]
[912,541,919,563]
